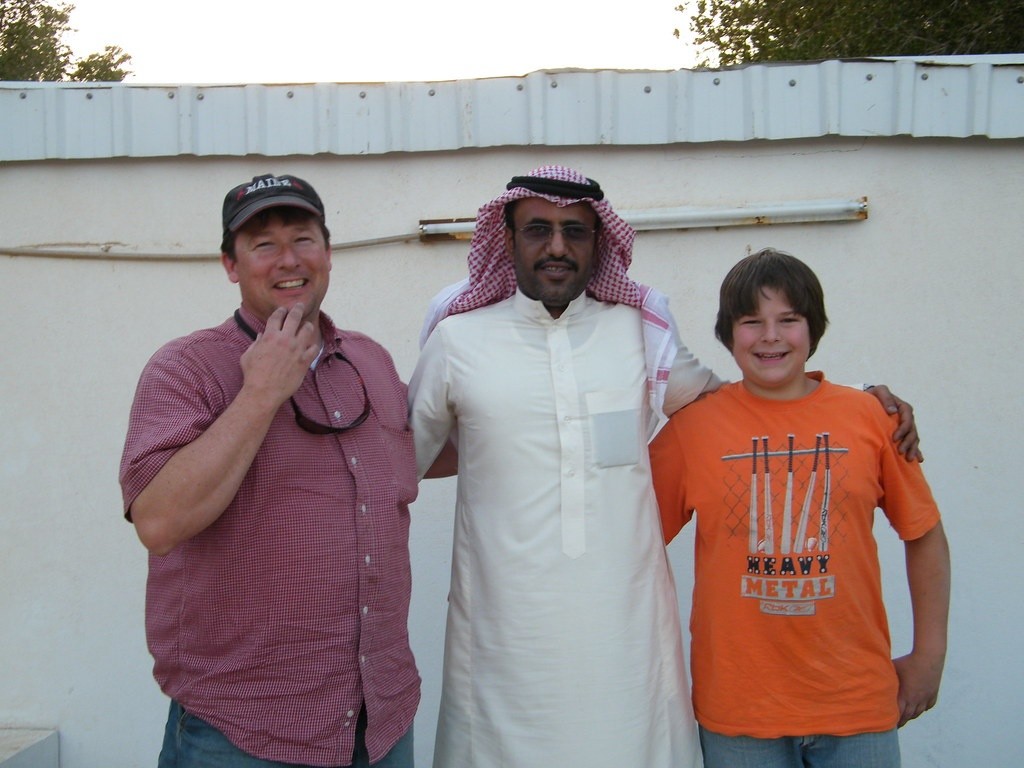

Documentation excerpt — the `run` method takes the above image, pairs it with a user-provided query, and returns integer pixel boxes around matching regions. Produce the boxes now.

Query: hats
[222,173,339,238]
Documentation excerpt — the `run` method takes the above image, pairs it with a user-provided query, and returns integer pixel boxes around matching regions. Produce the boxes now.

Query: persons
[648,248,951,768]
[407,167,926,767]
[119,174,463,768]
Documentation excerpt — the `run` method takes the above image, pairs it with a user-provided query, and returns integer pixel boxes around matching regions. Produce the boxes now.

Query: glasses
[288,351,372,435]
[514,223,599,242]
